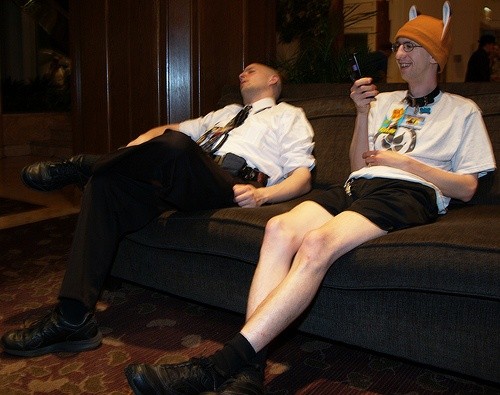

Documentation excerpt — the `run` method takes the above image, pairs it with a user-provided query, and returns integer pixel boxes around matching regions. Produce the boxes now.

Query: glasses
[391,41,423,54]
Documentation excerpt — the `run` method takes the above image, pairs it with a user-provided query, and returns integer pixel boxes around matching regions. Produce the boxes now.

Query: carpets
[1,201,500,394]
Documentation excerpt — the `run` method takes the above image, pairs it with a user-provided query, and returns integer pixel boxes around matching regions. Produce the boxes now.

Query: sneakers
[125,349,232,394]
[217,363,264,395]
[21,152,91,192]
[1,310,103,357]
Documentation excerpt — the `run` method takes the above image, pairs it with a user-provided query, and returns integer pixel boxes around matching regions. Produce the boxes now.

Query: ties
[200,105,252,151]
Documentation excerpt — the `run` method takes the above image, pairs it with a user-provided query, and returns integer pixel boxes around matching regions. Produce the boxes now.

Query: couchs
[116,86,500,385]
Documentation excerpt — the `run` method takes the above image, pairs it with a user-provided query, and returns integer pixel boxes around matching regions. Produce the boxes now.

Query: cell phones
[345,53,370,100]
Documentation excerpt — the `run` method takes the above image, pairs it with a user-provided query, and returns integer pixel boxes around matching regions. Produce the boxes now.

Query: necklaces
[406,83,440,107]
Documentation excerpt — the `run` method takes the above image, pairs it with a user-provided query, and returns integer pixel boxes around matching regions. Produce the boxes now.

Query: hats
[393,1,454,73]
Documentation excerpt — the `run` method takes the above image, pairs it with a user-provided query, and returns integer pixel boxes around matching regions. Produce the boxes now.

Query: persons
[124,1,497,395]
[0,63,317,359]
